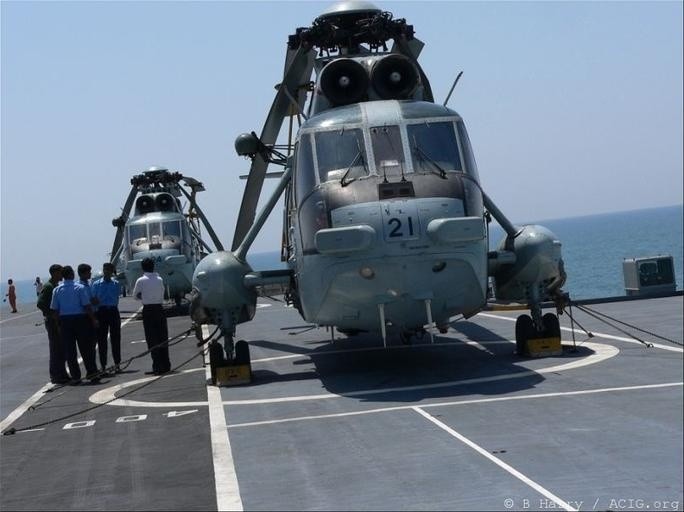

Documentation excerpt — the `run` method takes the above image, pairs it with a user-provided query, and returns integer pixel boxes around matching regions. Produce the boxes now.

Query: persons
[36,263,123,386]
[131,257,170,375]
[4,278,17,313]
[33,276,43,298]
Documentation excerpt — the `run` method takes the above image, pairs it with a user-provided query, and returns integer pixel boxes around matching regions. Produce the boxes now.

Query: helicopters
[91,165,224,308]
[189,1,569,388]
